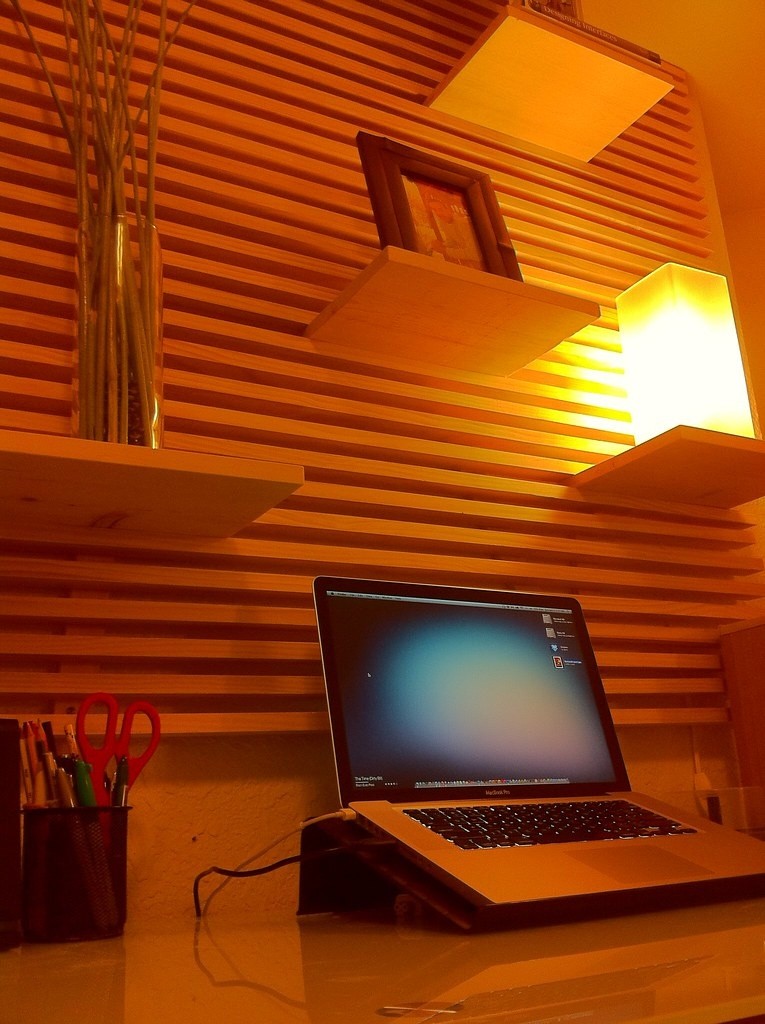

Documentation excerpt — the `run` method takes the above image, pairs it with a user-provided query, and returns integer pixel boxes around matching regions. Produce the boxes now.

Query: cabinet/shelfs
[2,7,765,527]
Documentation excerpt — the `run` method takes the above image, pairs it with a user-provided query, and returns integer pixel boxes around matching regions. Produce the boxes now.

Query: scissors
[77,693,160,859]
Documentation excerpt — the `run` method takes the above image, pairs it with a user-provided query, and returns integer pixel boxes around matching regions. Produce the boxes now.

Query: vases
[77,209,162,441]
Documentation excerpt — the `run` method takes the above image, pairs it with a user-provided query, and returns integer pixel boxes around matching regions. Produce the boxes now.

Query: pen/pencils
[19,718,129,933]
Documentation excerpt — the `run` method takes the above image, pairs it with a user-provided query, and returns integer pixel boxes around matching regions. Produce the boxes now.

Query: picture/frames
[352,130,525,283]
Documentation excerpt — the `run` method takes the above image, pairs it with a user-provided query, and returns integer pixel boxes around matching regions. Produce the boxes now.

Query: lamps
[616,264,755,445]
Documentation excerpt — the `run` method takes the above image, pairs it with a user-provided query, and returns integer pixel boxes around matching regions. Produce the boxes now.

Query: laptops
[313,574,765,909]
